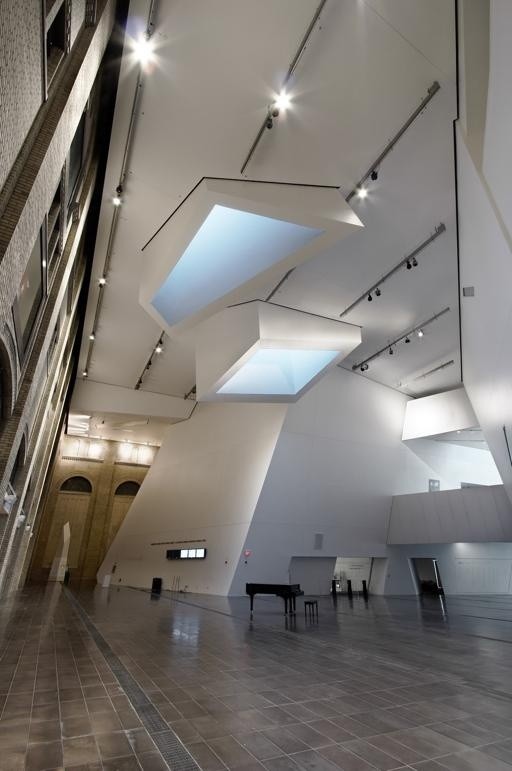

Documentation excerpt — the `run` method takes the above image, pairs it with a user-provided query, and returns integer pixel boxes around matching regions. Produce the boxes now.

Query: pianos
[246,583,304,614]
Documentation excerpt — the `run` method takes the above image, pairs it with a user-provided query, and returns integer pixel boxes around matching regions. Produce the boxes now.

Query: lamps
[366,256,419,303]
[352,328,435,372]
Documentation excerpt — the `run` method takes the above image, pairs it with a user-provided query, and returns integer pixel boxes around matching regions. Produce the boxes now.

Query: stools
[305,617,318,631]
[304,599,318,616]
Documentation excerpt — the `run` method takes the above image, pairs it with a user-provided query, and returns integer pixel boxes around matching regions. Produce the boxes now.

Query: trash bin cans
[151,577,162,595]
